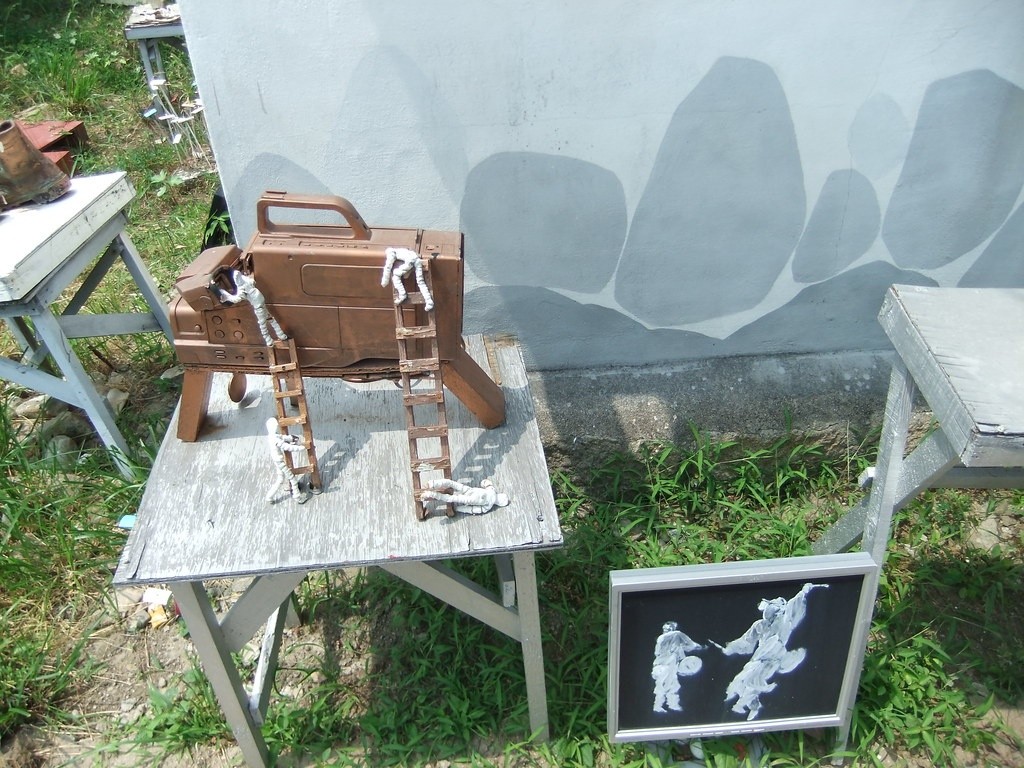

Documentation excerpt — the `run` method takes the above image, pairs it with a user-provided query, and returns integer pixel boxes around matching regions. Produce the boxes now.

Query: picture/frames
[607,551,879,744]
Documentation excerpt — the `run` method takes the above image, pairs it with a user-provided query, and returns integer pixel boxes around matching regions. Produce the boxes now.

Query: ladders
[389,259,457,521]
[265,338,322,491]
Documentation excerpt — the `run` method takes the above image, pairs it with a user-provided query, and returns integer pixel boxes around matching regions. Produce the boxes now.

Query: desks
[123,4,184,118]
[111,334,569,768]
[1,212,181,482]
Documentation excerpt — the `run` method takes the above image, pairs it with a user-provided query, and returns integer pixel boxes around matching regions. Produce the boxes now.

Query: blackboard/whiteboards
[607,551,881,745]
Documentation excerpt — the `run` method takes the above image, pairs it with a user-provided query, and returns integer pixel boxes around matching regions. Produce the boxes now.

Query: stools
[800,284,1023,767]
